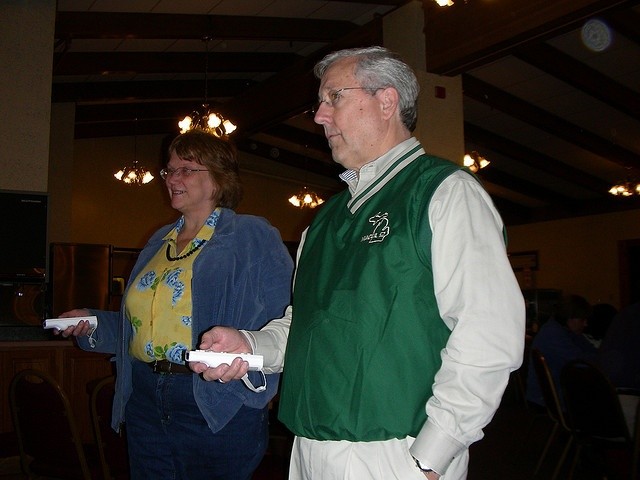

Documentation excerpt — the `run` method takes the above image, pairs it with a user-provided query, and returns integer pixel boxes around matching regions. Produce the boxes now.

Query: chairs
[515,353,628,479]
[558,358,640,479]
[7,368,91,480]
[90,375,131,479]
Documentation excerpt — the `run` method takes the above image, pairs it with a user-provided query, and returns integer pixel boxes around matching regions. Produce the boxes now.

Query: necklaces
[166,239,207,261]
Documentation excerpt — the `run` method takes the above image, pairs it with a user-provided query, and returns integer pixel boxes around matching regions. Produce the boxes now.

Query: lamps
[607,180,639,197]
[177,34,239,138]
[463,149,491,173]
[287,144,325,210]
[112,119,155,186]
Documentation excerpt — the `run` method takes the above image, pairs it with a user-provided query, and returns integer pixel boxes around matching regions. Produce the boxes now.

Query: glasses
[160,167,213,180]
[311,87,386,115]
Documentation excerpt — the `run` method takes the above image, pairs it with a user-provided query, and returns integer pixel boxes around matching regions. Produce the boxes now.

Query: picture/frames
[508,249,539,272]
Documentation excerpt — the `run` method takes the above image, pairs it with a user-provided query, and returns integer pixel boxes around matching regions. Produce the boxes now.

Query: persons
[524,297,536,318]
[53,131,295,480]
[188,46,526,480]
[580,303,639,441]
[525,318,541,338]
[526,294,599,416]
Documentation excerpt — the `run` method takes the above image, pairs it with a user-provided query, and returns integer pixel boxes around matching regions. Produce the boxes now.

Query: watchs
[412,457,438,474]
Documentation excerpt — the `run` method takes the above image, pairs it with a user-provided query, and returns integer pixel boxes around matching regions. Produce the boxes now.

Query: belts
[147,360,194,375]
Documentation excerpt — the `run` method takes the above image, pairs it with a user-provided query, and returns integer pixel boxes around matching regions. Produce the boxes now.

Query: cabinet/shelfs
[2,342,116,444]
